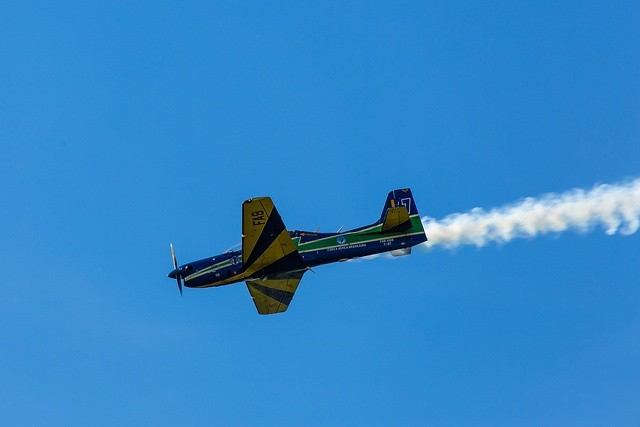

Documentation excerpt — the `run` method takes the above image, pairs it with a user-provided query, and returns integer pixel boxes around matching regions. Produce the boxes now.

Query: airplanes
[167,187,428,315]
